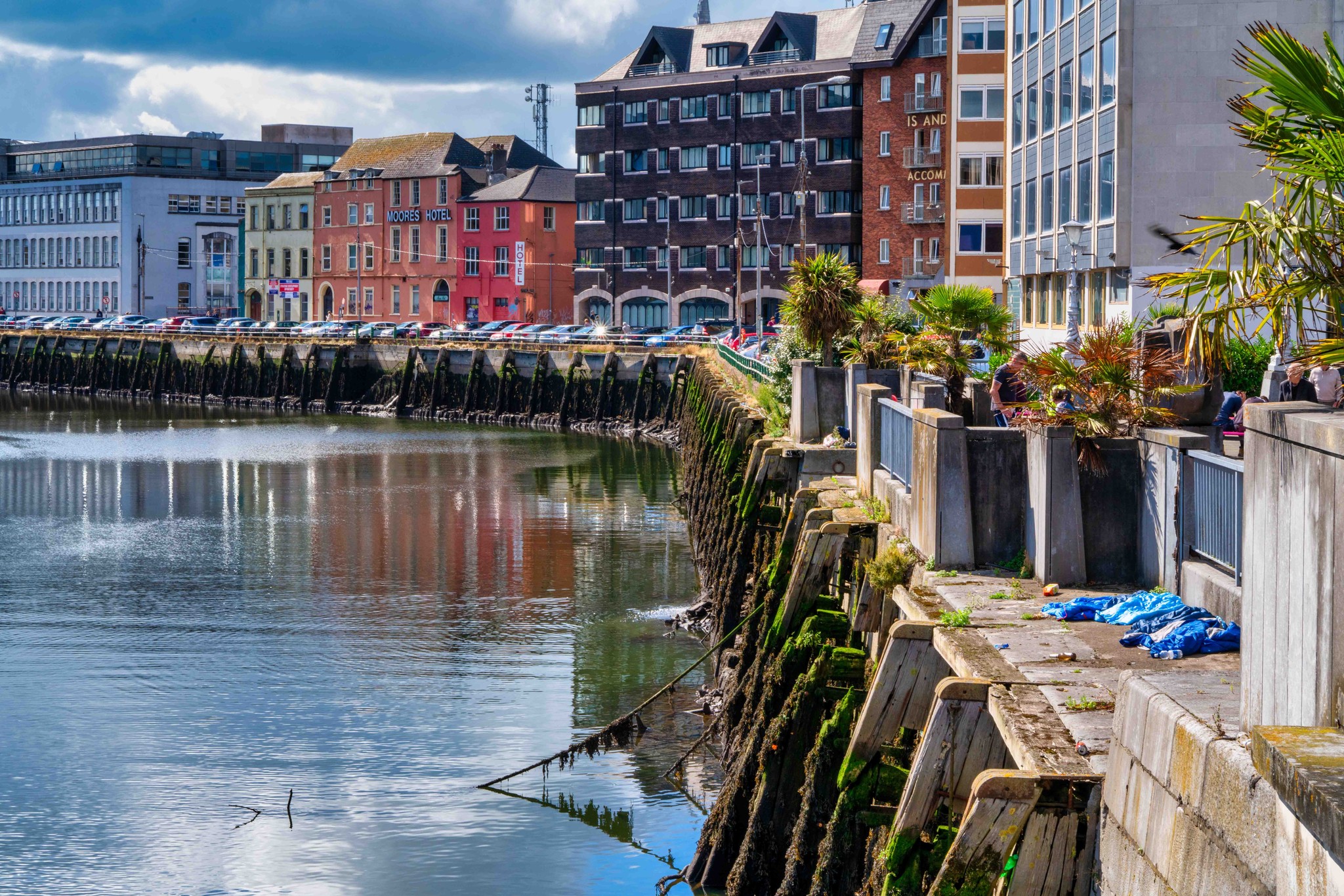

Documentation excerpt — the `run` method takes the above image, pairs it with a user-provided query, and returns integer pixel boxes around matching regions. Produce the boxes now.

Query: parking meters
[756,154,775,339]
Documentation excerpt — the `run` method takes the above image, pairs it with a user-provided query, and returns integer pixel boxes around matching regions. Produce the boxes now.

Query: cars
[732,334,783,367]
[130,319,165,334]
[710,328,732,346]
[249,320,694,347]
[0,314,113,335]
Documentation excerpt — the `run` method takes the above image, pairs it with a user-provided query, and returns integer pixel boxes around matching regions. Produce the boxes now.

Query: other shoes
[1223,447,1225,455]
[1238,449,1244,457]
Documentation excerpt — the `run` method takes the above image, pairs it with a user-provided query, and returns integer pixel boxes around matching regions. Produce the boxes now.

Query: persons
[206,309,219,320]
[96,309,103,318]
[584,319,590,327]
[733,315,737,321]
[589,311,599,326]
[326,311,334,321]
[1212,390,1269,457]
[990,352,1028,427]
[1052,388,1077,425]
[1278,363,1343,408]
[0,307,6,315]
[975,333,983,343]
[767,316,776,326]
[622,322,631,334]
[124,312,141,315]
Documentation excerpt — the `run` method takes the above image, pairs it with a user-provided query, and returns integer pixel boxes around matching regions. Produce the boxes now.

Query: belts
[1214,419,1219,421]
[1234,423,1242,425]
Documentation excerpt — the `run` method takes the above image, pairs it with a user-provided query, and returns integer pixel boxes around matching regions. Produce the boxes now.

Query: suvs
[180,317,220,335]
[959,339,988,361]
[691,318,748,347]
[468,320,523,341]
[730,325,776,347]
[162,316,193,334]
[216,317,256,337]
[110,315,149,332]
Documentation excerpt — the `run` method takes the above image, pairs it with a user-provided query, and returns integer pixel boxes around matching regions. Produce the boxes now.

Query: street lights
[736,179,753,326]
[658,191,672,328]
[134,214,146,316]
[1056,216,1090,368]
[799,75,850,262]
[347,202,361,320]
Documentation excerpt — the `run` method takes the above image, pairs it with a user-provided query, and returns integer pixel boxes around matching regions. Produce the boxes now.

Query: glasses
[1286,371,1302,374]
